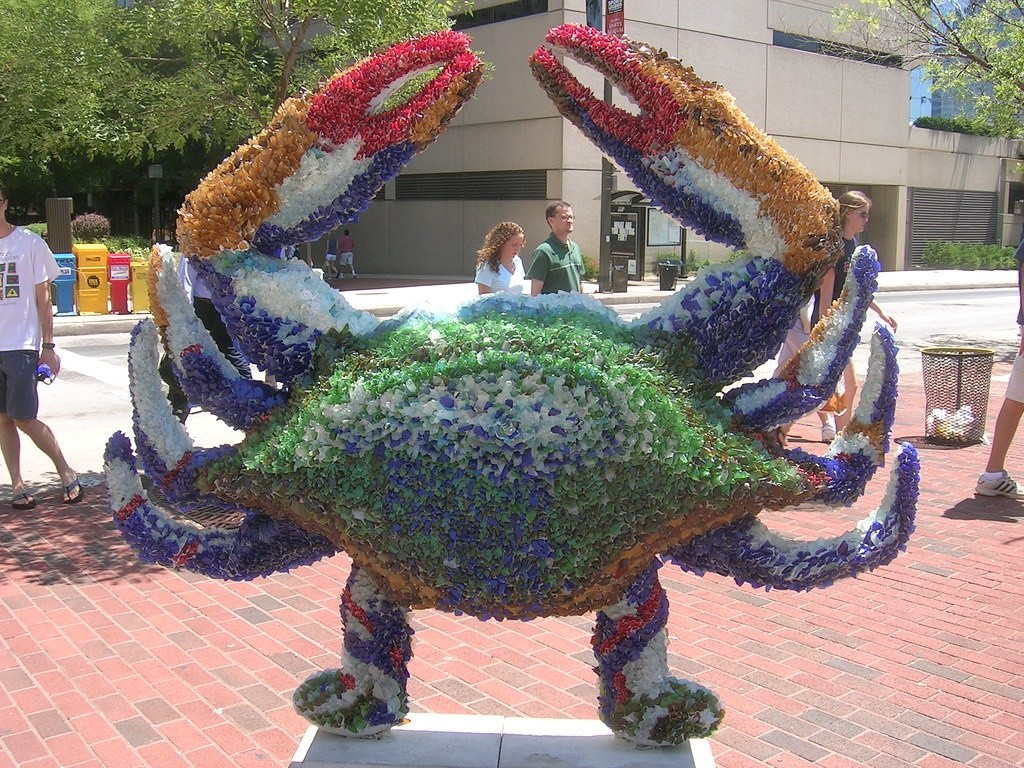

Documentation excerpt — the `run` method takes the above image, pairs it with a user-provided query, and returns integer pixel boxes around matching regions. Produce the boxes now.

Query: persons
[526,201,586,297]
[325,230,357,280]
[273,243,302,261]
[770,189,896,446]
[1,183,84,506]
[476,221,526,296]
[187,262,253,380]
[974,228,1023,499]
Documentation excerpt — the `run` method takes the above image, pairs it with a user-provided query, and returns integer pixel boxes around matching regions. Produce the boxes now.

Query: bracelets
[42,342,55,349]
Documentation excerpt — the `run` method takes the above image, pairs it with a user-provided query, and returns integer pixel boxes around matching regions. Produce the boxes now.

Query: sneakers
[975,471,1024,499]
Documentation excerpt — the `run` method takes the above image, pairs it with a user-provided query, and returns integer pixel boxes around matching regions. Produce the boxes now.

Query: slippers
[12,491,36,509]
[63,478,84,503]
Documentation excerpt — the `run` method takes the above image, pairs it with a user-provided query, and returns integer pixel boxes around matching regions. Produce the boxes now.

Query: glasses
[851,211,869,219]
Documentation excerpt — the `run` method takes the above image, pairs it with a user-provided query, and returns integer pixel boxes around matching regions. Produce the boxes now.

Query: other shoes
[822,418,836,442]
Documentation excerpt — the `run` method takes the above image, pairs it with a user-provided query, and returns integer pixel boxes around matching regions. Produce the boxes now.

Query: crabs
[102,23,920,747]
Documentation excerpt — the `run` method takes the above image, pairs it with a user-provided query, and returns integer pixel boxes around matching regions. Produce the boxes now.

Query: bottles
[37,354,61,381]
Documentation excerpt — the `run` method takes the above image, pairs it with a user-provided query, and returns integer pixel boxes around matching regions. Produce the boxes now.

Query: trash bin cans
[657,259,681,291]
[920,347,995,448]
[52,242,152,315]
